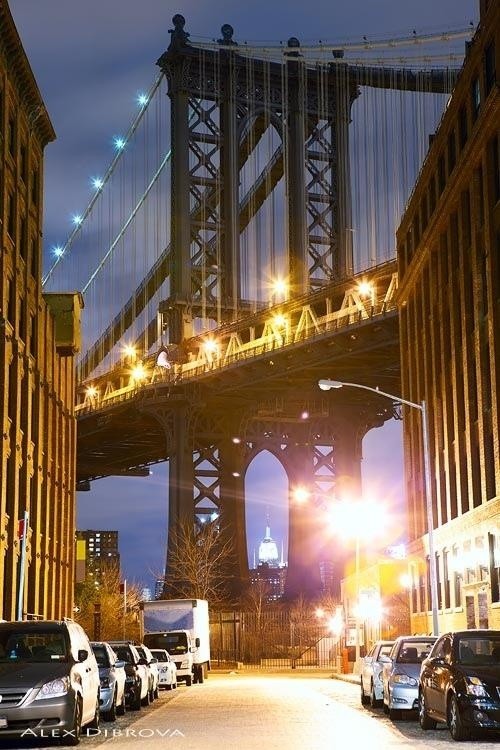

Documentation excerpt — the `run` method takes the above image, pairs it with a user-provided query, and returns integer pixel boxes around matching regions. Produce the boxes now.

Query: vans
[0,617,100,747]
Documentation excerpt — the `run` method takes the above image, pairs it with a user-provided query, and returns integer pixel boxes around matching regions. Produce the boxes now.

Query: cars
[361,626,500,745]
[45,640,177,723]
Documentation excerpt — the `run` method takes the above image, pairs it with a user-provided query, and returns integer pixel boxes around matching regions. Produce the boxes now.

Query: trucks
[142,598,211,686]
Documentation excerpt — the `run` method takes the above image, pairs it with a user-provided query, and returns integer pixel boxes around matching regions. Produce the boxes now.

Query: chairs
[401,647,417,663]
[4,638,63,657]
[461,646,499,659]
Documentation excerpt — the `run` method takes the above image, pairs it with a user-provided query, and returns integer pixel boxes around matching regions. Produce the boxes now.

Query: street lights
[318,379,440,637]
[294,490,362,677]
